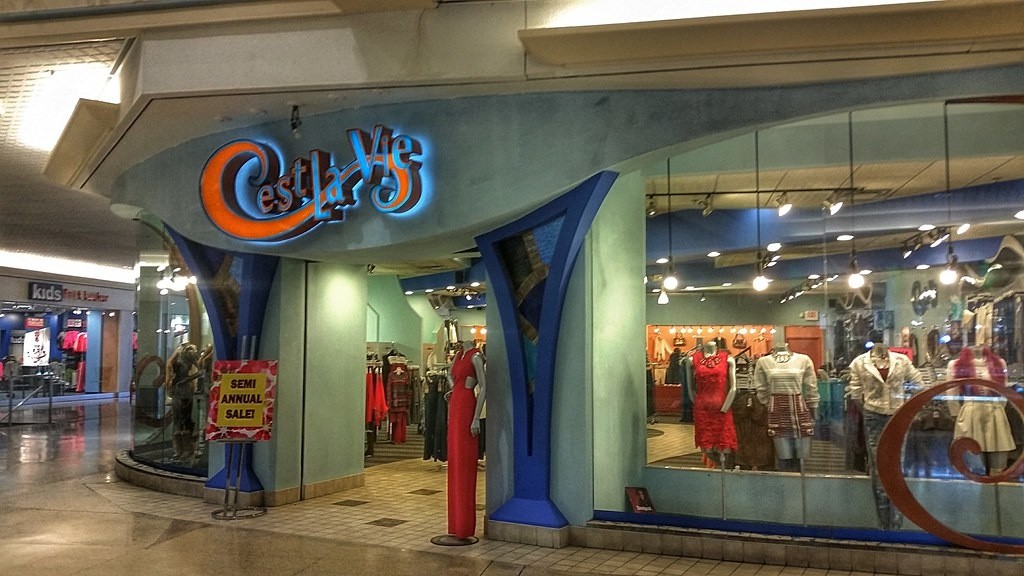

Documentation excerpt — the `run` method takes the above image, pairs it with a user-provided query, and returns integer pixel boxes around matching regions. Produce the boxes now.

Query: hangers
[366,346,406,366]
[425,364,450,379]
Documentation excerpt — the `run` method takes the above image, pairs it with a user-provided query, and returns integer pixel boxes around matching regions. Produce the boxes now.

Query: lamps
[753,130,769,291]
[820,197,843,215]
[700,292,706,302]
[653,324,660,335]
[680,325,687,333]
[848,112,864,289]
[657,285,670,304]
[687,325,694,333]
[645,197,657,214]
[697,194,715,217]
[669,325,676,334]
[779,273,840,305]
[900,222,969,258]
[771,192,792,217]
[938,101,959,284]
[403,283,483,310]
[852,258,872,275]
[663,158,679,290]
[697,324,703,334]
[719,324,776,334]
[707,325,714,333]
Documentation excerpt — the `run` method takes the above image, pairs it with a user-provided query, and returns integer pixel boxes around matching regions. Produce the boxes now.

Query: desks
[903,429,984,478]
